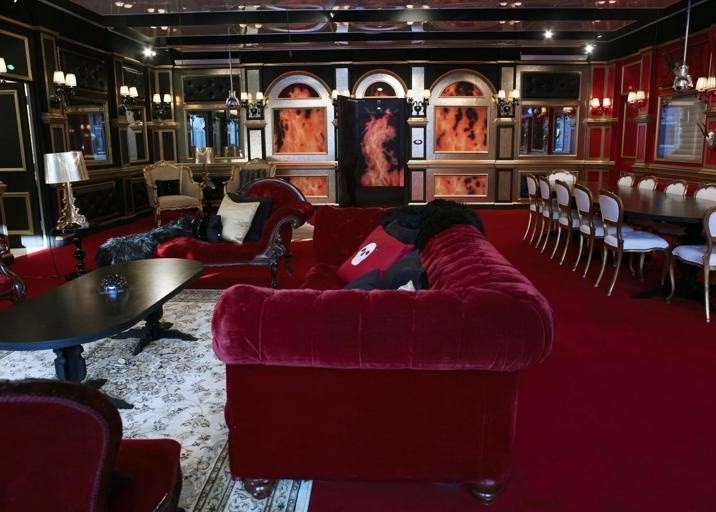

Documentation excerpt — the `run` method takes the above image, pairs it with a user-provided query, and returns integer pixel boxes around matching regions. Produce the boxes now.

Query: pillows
[194,192,273,243]
[336,220,427,293]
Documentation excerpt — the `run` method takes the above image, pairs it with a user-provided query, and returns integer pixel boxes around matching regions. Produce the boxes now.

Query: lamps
[43,150,90,230]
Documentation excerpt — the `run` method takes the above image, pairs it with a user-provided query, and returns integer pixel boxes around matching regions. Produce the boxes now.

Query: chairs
[157,178,315,288]
[524,173,716,323]
[0,381,183,512]
[142,157,277,226]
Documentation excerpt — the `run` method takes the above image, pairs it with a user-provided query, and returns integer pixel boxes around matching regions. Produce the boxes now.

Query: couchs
[212,201,556,500]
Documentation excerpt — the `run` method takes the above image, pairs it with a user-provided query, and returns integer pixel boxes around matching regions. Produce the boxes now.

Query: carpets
[0,289,313,512]
